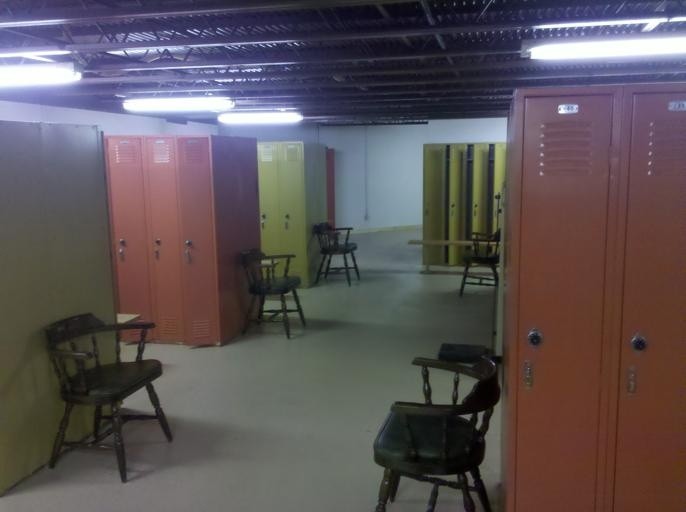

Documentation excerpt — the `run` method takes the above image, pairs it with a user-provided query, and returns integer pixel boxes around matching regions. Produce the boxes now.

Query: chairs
[459,228,501,296]
[314,221,360,287]
[42,313,173,484]
[373,342,502,510]
[240,247,308,339]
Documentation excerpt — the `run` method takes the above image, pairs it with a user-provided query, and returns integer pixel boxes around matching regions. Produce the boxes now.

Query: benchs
[407,239,500,275]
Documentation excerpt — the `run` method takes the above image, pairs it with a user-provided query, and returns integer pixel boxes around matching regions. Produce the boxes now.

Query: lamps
[220,110,304,128]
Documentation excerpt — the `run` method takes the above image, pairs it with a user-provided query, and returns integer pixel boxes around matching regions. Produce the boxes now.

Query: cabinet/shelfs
[254,138,335,288]
[498,80,686,512]
[102,132,262,349]
[422,142,507,268]
[0,118,120,491]
